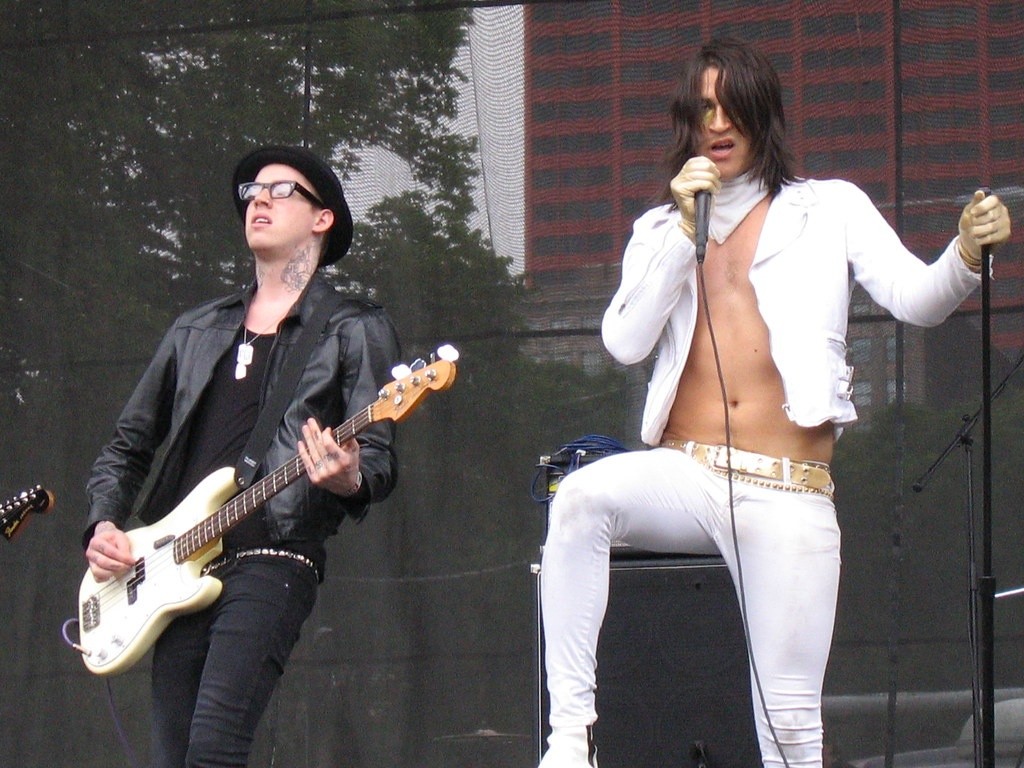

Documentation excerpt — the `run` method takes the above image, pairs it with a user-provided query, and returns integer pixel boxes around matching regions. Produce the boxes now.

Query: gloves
[670,156,721,245]
[955,190,1011,273]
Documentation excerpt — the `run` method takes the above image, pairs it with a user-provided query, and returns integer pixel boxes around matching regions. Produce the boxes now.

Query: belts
[200,548,319,585]
[661,439,832,490]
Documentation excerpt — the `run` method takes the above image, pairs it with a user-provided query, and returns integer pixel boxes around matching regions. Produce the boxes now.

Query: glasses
[238,181,326,210]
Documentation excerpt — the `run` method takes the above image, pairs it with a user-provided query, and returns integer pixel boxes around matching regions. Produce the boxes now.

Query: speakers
[527,556,764,768]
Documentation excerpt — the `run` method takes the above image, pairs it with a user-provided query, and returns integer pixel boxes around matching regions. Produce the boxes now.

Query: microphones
[691,189,712,262]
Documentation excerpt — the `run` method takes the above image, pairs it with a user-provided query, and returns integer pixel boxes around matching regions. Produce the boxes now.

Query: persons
[538,39,1010,768]
[82,146,405,768]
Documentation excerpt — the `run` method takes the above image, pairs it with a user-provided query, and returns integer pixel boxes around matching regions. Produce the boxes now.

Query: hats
[232,145,353,268]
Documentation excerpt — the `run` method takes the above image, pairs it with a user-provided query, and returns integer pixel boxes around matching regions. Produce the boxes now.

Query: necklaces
[233,316,282,379]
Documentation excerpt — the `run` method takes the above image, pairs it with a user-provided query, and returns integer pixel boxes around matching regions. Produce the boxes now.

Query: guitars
[0,483,56,544]
[75,341,461,681]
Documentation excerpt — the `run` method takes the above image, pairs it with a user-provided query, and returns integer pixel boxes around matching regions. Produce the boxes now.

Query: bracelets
[342,472,362,497]
